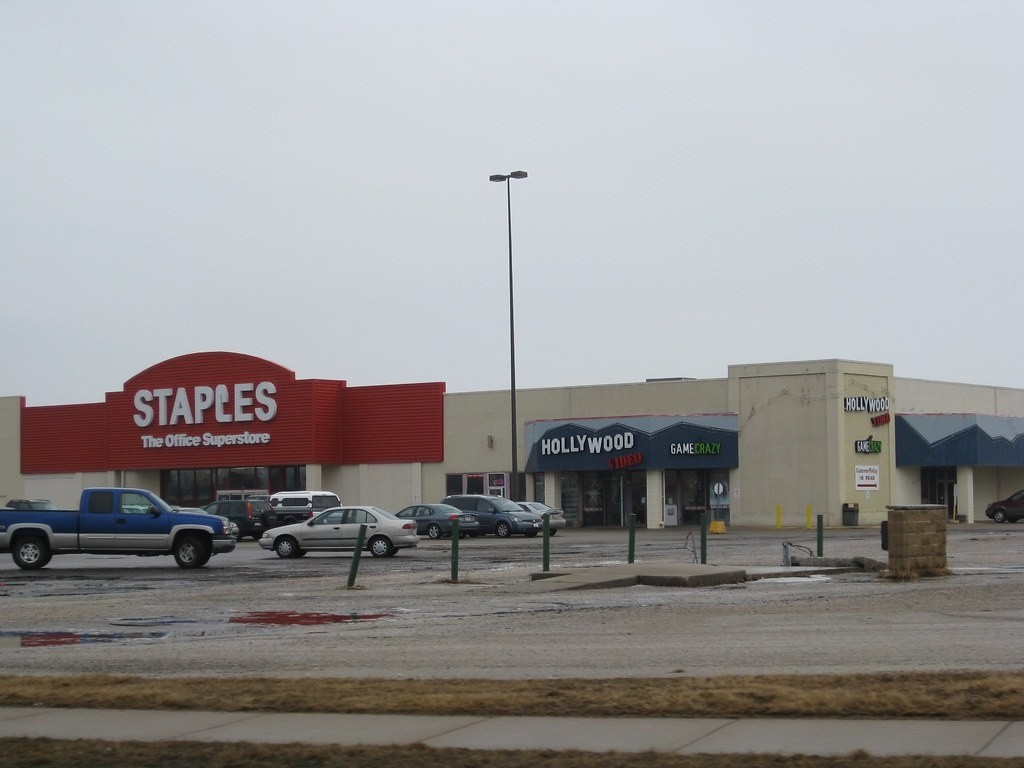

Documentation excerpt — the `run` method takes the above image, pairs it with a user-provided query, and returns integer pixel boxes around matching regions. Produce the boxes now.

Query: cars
[396,503,480,539]
[985,489,1023,523]
[5,497,57,511]
[258,506,419,559]
[439,495,542,539]
[515,500,566,536]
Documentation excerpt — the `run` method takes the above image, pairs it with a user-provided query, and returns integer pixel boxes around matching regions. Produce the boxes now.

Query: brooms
[948,496,959,524]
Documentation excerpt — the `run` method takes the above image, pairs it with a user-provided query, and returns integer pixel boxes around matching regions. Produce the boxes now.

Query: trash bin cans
[842,503,860,526]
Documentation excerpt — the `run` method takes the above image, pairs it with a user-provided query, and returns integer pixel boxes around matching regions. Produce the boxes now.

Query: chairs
[418,510,425,516]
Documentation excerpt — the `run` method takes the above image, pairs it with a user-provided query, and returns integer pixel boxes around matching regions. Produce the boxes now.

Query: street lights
[489,170,528,502]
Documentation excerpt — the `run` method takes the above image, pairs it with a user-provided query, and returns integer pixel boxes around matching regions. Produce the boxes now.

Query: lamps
[487,436,492,448]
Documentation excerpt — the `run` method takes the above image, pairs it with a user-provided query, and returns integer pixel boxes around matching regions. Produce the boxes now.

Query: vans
[270,491,342,509]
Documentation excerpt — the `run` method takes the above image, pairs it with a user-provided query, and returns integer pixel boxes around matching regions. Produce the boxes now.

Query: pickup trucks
[0,487,239,570]
[202,499,313,542]
